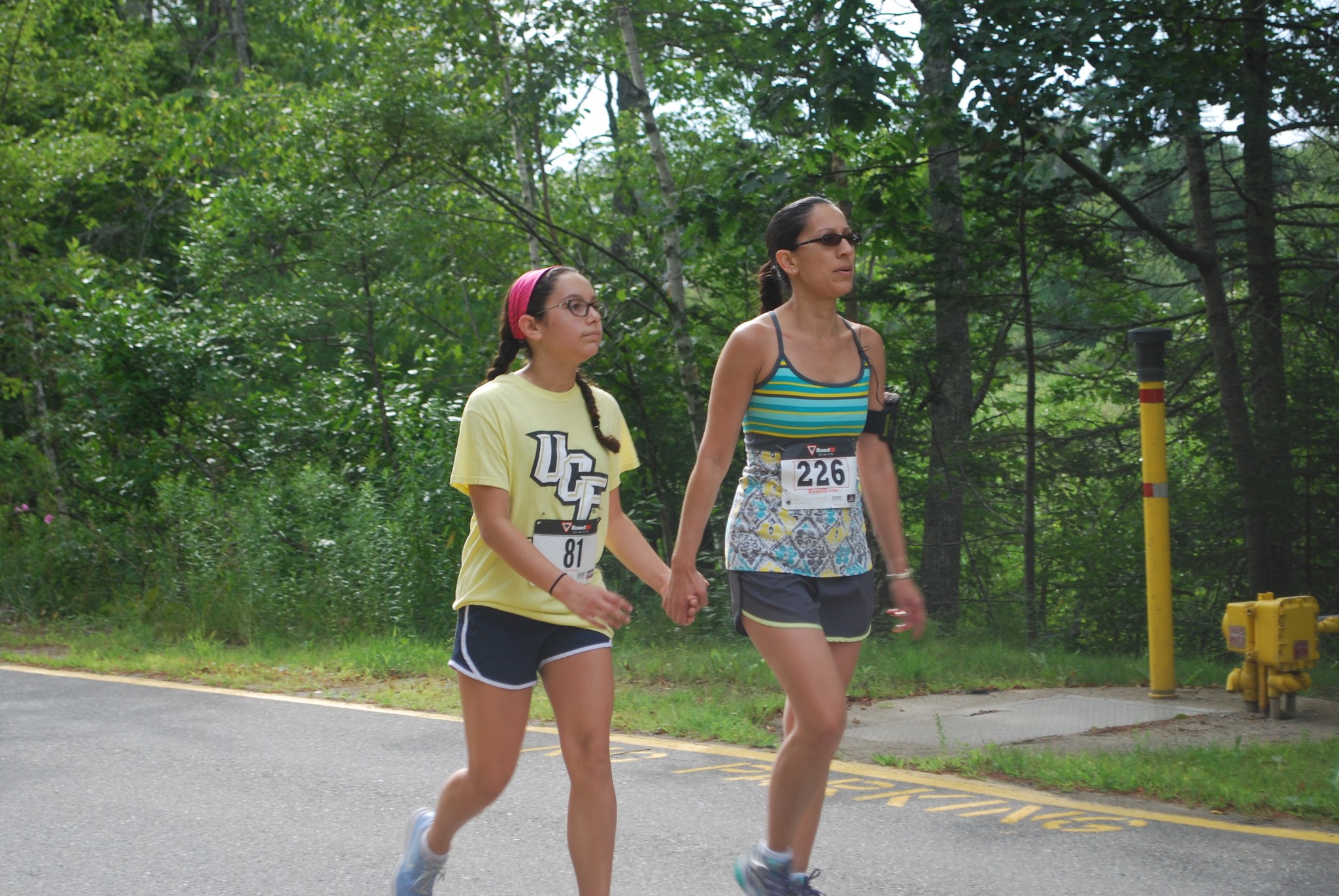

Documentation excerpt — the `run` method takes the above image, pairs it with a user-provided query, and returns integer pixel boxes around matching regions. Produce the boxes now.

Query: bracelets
[885,569,916,581]
[549,572,568,596]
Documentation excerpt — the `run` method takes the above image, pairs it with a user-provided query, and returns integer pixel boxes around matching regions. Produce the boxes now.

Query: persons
[390,266,710,895]
[661,195,923,896]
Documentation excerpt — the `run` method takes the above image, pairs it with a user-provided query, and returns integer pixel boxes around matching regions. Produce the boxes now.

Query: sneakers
[735,849,823,896]
[393,808,448,896]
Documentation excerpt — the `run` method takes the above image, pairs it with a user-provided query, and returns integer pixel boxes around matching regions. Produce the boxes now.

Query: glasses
[533,300,609,319]
[790,233,863,252]
